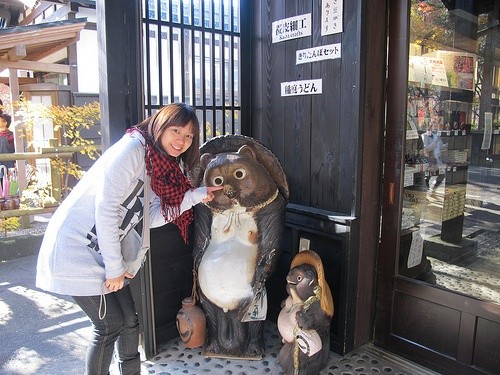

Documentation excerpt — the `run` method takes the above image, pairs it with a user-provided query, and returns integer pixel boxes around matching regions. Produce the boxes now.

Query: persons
[0,136,14,191]
[420,120,448,205]
[0,114,16,168]
[36,102,225,375]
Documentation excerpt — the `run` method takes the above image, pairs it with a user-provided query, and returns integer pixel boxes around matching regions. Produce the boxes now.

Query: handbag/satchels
[121,169,150,286]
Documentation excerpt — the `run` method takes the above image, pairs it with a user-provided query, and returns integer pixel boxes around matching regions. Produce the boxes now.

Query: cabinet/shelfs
[407,77,500,238]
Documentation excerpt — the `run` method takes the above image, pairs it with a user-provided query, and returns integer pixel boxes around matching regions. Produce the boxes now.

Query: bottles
[176,299,205,348]
